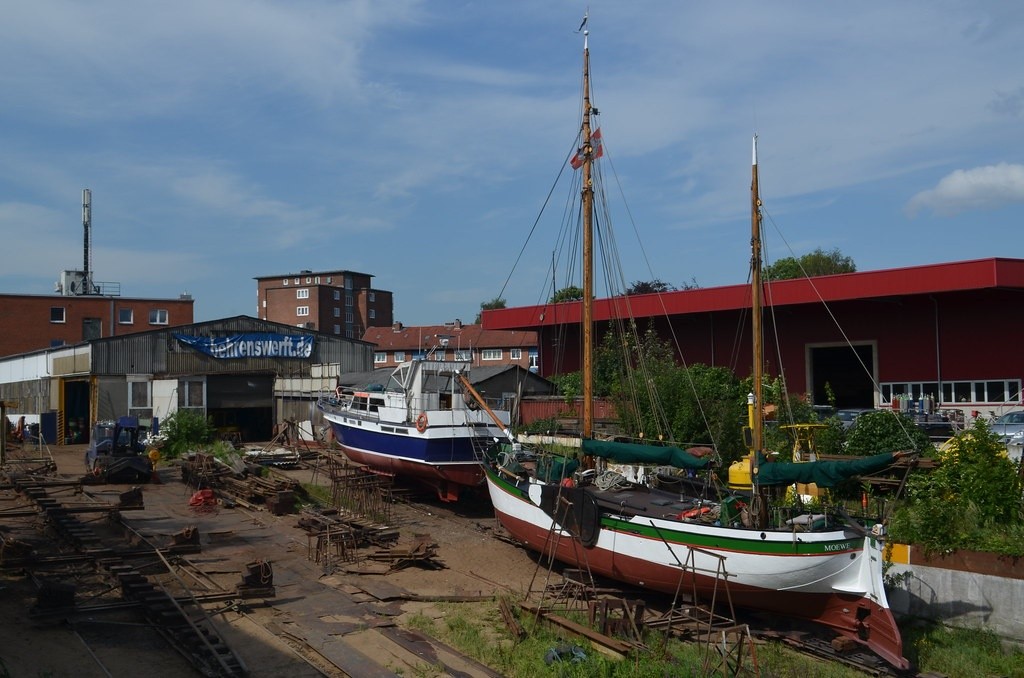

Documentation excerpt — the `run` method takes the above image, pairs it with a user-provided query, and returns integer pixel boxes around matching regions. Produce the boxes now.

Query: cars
[988,410,1024,447]
[832,408,876,432]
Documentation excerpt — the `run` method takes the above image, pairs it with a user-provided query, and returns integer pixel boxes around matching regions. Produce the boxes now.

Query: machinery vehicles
[83,414,158,486]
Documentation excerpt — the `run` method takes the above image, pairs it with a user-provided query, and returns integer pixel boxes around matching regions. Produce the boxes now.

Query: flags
[570,127,603,170]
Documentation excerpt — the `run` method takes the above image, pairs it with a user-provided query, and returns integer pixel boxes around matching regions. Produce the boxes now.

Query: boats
[314,324,514,489]
[454,13,924,672]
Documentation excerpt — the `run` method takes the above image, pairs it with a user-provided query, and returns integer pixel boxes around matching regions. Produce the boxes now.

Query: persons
[18,416,25,443]
[281,419,290,446]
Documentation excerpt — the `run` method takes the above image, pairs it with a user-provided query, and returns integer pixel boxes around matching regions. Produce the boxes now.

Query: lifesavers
[683,507,711,518]
[415,413,427,432]
